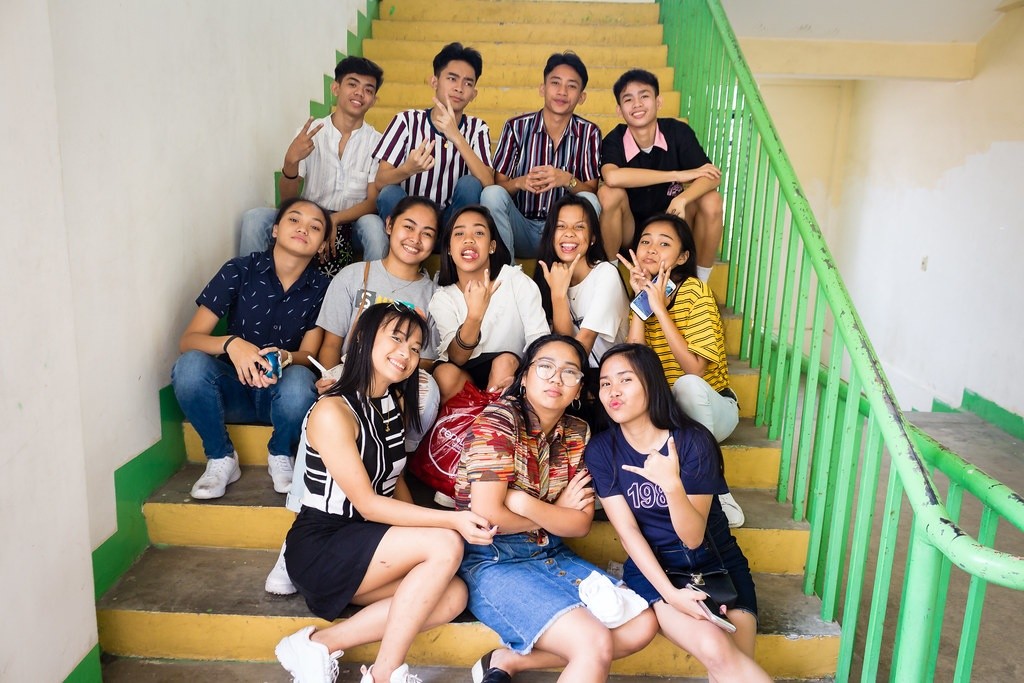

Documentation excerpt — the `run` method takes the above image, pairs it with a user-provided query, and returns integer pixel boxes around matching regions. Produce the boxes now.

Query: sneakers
[265,540,297,594]
[359,663,422,683]
[267,452,294,493]
[190,450,241,499]
[275,627,344,683]
[717,491,744,528]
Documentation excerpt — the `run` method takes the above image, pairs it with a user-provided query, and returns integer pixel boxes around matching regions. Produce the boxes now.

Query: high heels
[471,649,512,683]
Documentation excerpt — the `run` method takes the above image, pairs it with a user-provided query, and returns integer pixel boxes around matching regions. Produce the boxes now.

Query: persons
[616,214,744,530]
[476,51,602,266]
[369,40,495,228]
[427,204,552,405]
[169,195,331,499]
[531,198,632,423]
[582,341,775,682]
[597,68,724,287]
[449,334,659,683]
[272,303,470,683]
[236,54,387,264]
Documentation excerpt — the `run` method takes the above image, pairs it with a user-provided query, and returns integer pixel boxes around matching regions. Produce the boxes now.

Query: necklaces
[369,396,391,433]
[384,269,415,293]
[568,284,580,301]
[265,195,440,595]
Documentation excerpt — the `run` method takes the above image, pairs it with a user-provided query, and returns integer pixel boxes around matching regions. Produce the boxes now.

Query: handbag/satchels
[408,381,502,498]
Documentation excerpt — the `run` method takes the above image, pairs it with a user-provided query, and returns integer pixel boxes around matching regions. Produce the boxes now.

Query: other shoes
[434,491,455,506]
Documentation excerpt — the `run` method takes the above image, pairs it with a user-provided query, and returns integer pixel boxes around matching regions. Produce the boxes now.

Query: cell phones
[255,351,282,379]
[686,582,737,635]
[628,274,677,321]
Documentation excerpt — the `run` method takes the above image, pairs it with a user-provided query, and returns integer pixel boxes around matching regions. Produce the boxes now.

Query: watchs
[564,176,577,190]
[281,351,292,369]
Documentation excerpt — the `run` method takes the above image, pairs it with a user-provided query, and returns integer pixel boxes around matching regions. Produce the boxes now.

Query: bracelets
[224,335,238,352]
[281,168,299,179]
[455,323,482,350]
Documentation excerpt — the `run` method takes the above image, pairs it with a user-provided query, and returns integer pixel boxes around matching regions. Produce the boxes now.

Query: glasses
[527,359,585,388]
[385,300,418,315]
